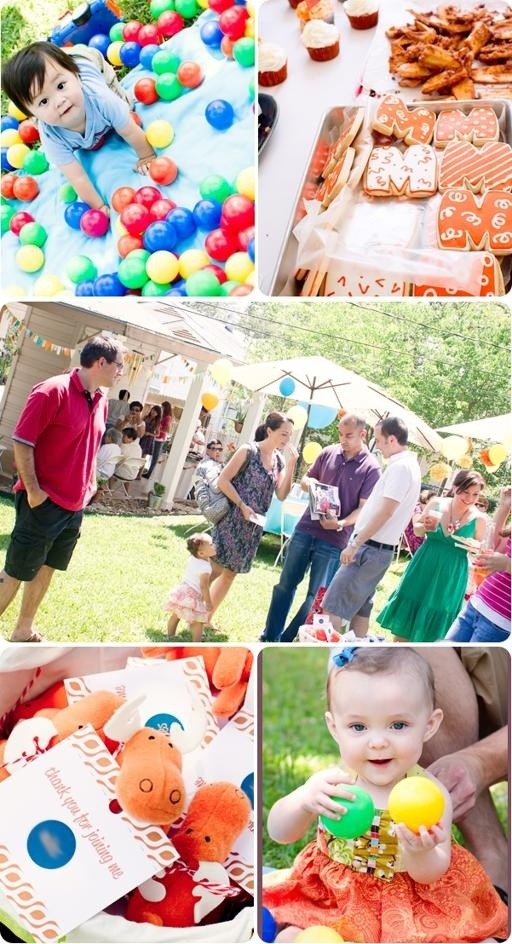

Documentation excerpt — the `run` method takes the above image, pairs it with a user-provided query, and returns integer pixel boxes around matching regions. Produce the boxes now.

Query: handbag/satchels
[194,468,230,527]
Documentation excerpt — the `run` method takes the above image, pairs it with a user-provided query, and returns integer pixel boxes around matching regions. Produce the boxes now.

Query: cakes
[300,19,339,62]
[258,38,287,85]
[343,0,378,30]
[295,0,335,25]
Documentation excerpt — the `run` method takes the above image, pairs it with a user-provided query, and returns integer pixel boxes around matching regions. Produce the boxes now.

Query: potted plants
[229,410,248,433]
[147,481,167,511]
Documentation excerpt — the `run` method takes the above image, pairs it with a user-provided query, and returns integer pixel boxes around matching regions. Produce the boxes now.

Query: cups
[301,25,340,62]
[345,6,381,31]
[259,58,288,87]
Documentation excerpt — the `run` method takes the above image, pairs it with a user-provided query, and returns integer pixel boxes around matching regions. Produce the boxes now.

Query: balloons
[200,391,218,410]
[279,378,295,396]
[287,406,308,430]
[489,444,505,464]
[505,434,511,450]
[440,436,469,460]
[481,449,496,465]
[379,453,392,464]
[484,463,501,474]
[302,442,321,464]
[432,464,451,480]
[210,358,235,385]
[299,401,338,428]
[456,455,472,469]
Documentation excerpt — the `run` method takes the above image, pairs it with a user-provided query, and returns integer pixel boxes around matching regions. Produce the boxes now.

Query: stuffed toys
[206,99,233,131]
[134,78,159,104]
[319,785,376,841]
[294,925,343,944]
[171,252,180,283]
[202,264,227,285]
[126,783,251,924]
[141,279,171,297]
[19,222,49,248]
[248,73,255,98]
[9,100,28,121]
[163,289,188,296]
[24,150,47,174]
[106,41,126,66]
[150,156,178,186]
[200,174,232,202]
[120,203,149,233]
[261,905,280,941]
[207,0,234,14]
[172,279,186,289]
[16,246,44,272]
[247,272,256,286]
[130,111,141,127]
[92,274,127,295]
[6,143,31,168]
[1,689,127,787]
[205,228,238,261]
[79,209,109,238]
[166,207,197,239]
[10,211,35,236]
[138,44,161,71]
[1,205,16,231]
[3,285,24,298]
[177,60,204,88]
[0,128,23,146]
[67,255,97,283]
[139,234,142,247]
[174,0,200,18]
[34,273,65,297]
[60,183,77,203]
[136,186,163,210]
[119,257,149,289]
[158,11,185,37]
[146,120,174,148]
[197,0,209,11]
[193,198,222,231]
[128,289,142,296]
[222,194,255,226]
[145,250,179,283]
[388,777,444,835]
[1,115,18,134]
[237,226,255,252]
[112,186,135,213]
[116,215,143,236]
[185,271,220,296]
[248,238,255,263]
[252,100,256,114]
[221,33,235,58]
[119,40,141,67]
[125,248,151,261]
[245,17,255,39]
[235,166,255,201]
[247,1,255,18]
[113,272,118,277]
[1,196,8,205]
[17,119,41,144]
[219,8,248,39]
[149,199,176,222]
[219,280,241,297]
[76,280,96,296]
[230,284,255,297]
[123,20,142,41]
[109,23,125,42]
[1,147,17,171]
[154,73,182,100]
[150,0,175,20]
[179,249,209,280]
[232,38,255,67]
[108,726,187,825]
[0,174,18,199]
[117,234,141,257]
[152,50,179,76]
[63,202,91,230]
[225,252,255,283]
[233,0,246,6]
[144,647,252,720]
[88,33,112,59]
[143,222,177,251]
[62,291,73,296]
[139,24,163,46]
[13,176,39,201]
[201,20,224,47]
[220,214,232,230]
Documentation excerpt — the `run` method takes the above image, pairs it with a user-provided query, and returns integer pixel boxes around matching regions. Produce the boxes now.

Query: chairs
[273,499,309,569]
[95,454,126,496]
[111,456,147,496]
[396,531,415,561]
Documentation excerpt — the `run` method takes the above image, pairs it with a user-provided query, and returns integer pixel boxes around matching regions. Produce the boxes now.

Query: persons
[445,486,511,640]
[167,531,212,640]
[115,428,143,481]
[97,428,121,482]
[117,401,145,439]
[192,406,210,451]
[474,496,489,513]
[259,416,381,642]
[194,437,236,488]
[140,405,161,476]
[258,647,511,944]
[106,390,130,427]
[233,420,242,440]
[377,470,486,641]
[0,44,158,217]
[0,336,126,641]
[404,489,439,556]
[408,646,510,912]
[141,400,172,479]
[319,418,423,637]
[209,412,298,632]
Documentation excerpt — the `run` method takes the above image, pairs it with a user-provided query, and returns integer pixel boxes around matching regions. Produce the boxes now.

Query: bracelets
[235,499,245,508]
[348,537,362,550]
[502,553,511,574]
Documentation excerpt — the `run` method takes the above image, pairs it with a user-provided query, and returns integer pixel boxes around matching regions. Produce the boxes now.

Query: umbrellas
[435,411,512,452]
[230,353,445,494]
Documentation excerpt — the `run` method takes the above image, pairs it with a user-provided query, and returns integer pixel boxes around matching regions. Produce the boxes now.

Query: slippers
[9,632,47,642]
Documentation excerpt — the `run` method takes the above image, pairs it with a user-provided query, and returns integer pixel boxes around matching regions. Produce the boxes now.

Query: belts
[354,534,395,550]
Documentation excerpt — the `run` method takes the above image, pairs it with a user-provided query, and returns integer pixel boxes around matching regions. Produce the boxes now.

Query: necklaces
[449,499,471,528]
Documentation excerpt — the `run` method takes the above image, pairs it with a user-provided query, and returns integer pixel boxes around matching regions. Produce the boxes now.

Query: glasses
[130,408,140,412]
[475,503,485,507]
[210,447,223,452]
[113,361,122,369]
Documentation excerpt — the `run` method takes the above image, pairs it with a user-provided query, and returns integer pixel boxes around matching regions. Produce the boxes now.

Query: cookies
[292,98,512,295]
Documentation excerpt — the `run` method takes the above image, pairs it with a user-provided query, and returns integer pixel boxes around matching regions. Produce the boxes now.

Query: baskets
[298,624,368,643]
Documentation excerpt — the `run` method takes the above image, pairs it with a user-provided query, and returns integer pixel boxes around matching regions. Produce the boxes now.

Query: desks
[263,484,316,535]
